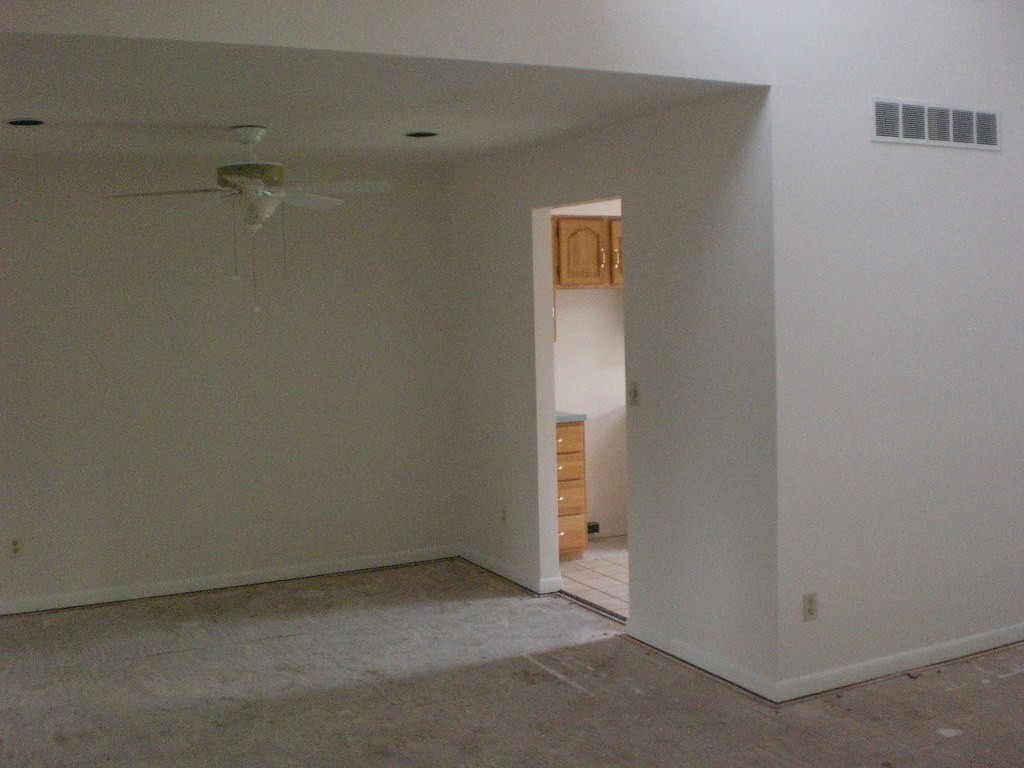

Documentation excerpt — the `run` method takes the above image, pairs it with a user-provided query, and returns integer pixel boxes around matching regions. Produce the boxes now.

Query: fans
[107,126,391,216]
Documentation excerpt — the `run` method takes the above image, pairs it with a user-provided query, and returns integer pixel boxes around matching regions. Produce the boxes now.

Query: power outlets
[803,593,818,619]
[588,522,600,534]
[7,536,22,558]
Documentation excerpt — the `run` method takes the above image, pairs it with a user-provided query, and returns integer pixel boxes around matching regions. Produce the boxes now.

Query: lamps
[230,199,282,236]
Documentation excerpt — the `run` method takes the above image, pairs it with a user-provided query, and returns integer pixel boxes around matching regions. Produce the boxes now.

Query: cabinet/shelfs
[556,412,588,561]
[553,214,621,285]
